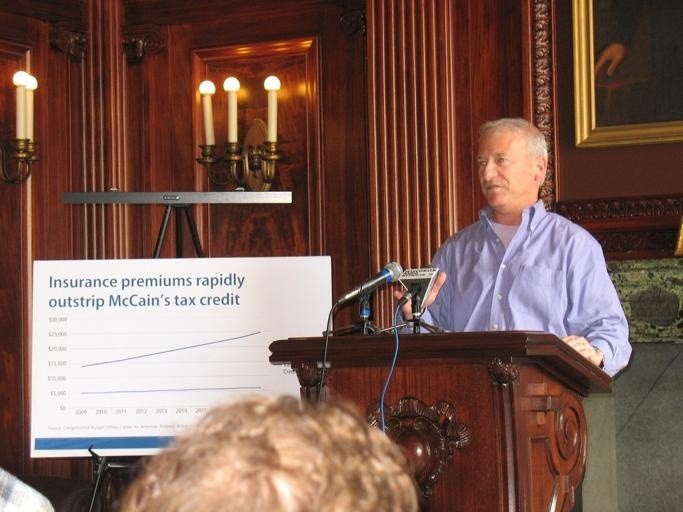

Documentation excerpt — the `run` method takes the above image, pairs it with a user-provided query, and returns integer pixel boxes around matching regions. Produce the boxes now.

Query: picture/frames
[572,0,683,147]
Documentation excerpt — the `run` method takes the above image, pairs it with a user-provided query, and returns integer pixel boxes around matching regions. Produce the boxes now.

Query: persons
[592,0,675,80]
[394,117,632,379]
[117,393,422,511]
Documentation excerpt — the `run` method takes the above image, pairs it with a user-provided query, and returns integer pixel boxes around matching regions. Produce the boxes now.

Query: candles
[254,74,281,192]
[220,75,244,189]
[3,69,25,186]
[12,75,38,187]
[196,77,221,189]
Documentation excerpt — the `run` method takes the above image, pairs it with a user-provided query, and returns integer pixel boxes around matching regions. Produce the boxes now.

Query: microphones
[336,258,404,307]
[396,265,440,318]
